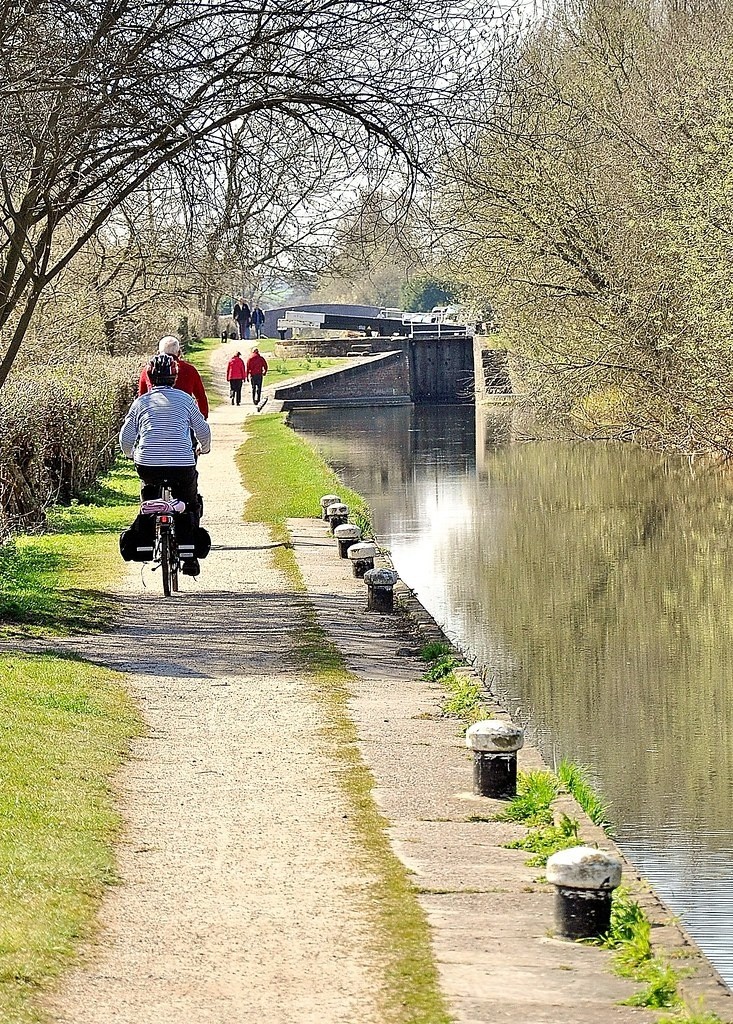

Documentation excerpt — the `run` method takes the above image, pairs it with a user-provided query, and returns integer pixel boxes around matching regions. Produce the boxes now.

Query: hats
[236,351,241,355]
[252,347,259,352]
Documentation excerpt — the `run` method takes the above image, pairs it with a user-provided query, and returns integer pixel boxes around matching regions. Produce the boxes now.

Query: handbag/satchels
[119,515,156,563]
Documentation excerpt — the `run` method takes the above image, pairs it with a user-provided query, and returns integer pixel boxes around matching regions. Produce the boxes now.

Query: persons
[226,351,246,405]
[246,348,269,404]
[233,297,266,340]
[119,336,212,577]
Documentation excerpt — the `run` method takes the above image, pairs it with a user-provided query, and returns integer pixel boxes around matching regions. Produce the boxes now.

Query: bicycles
[120,447,209,595]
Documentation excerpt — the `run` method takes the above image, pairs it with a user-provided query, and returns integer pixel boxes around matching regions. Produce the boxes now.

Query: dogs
[221,323,231,344]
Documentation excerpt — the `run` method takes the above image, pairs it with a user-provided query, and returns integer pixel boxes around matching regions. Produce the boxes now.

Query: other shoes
[237,404,240,406]
[258,397,260,402]
[183,558,200,576]
[231,398,234,405]
[254,400,255,404]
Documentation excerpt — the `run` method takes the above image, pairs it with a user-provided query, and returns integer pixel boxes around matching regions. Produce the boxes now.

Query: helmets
[146,353,179,385]
